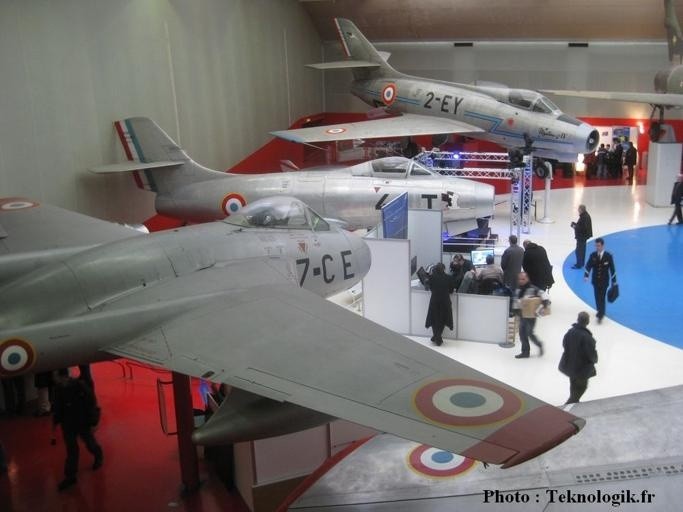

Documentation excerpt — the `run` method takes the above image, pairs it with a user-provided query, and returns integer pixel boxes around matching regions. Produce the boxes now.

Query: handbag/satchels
[608,285,618,302]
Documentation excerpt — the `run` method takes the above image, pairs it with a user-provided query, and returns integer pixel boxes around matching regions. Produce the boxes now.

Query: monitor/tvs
[470,248,495,267]
[417,266,428,286]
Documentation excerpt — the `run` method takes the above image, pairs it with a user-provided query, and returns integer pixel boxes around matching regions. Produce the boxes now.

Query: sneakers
[515,354,529,358]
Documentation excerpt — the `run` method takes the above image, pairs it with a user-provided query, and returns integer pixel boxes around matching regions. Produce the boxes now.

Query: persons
[582,237,618,322]
[557,310,597,404]
[415,235,555,360]
[570,205,593,271]
[46,367,104,493]
[666,173,683,226]
[583,136,638,181]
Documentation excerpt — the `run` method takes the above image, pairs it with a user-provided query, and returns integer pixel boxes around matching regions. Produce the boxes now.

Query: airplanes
[269,16,604,181]
[88,114,501,240]
[538,0,683,139]
[0,189,591,477]
[286,381,682,512]
